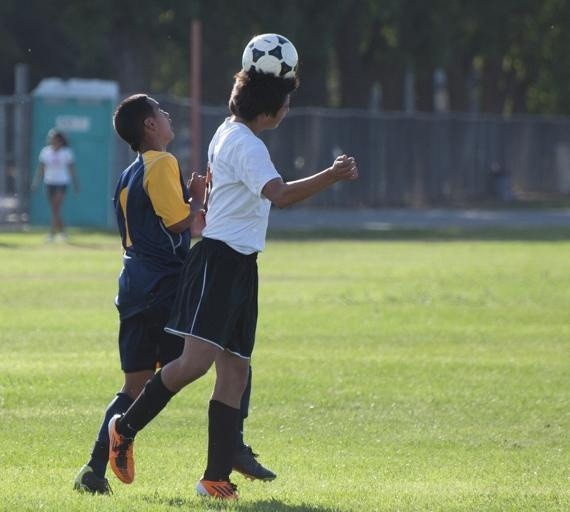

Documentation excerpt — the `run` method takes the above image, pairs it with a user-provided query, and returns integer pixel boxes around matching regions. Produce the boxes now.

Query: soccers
[242,33,299,79]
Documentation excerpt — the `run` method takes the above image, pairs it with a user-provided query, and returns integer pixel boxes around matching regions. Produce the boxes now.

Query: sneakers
[107,413,134,484]
[196,480,240,500]
[229,444,277,482]
[72,464,112,497]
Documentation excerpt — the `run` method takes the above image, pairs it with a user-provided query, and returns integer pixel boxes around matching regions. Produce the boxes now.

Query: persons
[68,91,277,495]
[31,128,79,242]
[108,70,359,499]
